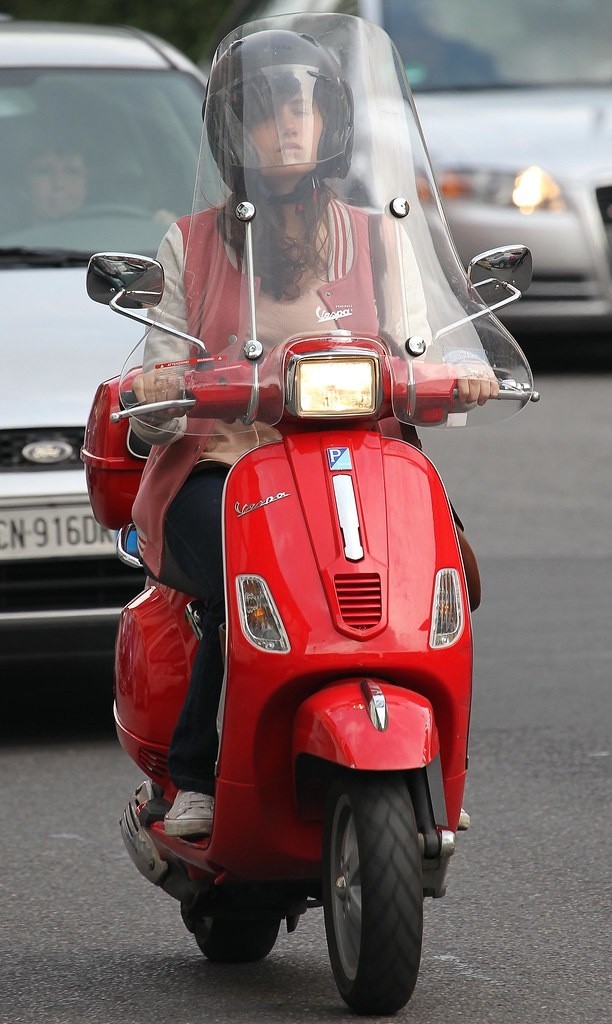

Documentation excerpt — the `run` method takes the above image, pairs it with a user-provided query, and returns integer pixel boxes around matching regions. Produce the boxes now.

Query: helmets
[201,29,352,190]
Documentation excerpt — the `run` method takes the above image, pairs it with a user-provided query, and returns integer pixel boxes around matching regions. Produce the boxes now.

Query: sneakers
[456,810,468,829]
[163,789,213,836]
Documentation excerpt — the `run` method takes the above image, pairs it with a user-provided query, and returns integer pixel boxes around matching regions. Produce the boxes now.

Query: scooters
[83,11,547,1013]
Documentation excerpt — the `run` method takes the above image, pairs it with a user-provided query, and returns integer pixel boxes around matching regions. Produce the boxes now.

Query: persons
[13,132,181,232]
[128,29,500,839]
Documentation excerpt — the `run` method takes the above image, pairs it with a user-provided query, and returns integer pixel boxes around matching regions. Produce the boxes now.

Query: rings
[156,376,165,380]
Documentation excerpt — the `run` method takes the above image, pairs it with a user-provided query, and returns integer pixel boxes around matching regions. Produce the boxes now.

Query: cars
[0,19,229,662]
[255,0,609,373]
